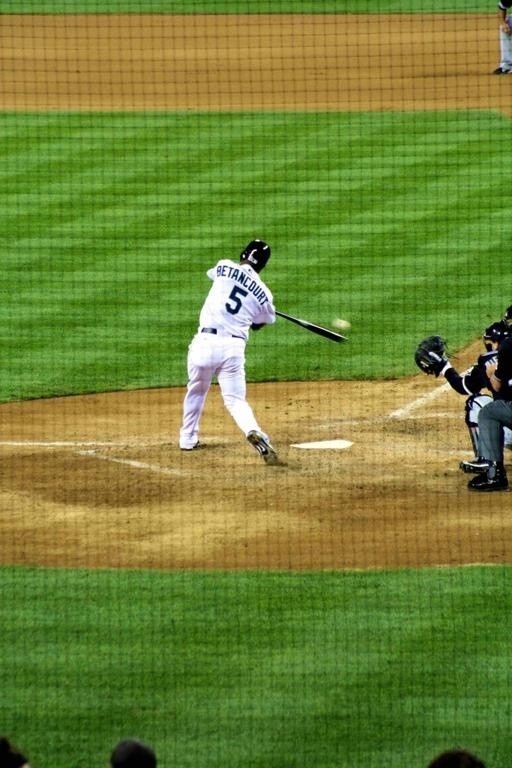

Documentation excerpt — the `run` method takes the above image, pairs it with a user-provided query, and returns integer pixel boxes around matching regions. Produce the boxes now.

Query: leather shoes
[467,477,509,493]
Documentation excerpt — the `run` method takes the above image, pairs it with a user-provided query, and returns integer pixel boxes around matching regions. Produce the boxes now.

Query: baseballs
[332,318,351,330]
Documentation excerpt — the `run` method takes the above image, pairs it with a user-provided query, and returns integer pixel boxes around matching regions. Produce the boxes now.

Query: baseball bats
[275,311,348,344]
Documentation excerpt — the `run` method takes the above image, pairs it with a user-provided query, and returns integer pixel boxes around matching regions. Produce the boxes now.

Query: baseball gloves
[415,336,448,377]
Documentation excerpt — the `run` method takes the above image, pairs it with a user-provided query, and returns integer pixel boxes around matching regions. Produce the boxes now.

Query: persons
[1,736,28,768]
[429,750,485,768]
[495,0,511,75]
[110,740,155,768]
[417,302,512,491]
[460,321,512,473]
[179,238,283,463]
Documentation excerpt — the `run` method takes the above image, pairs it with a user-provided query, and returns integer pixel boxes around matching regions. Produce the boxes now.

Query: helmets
[482,320,510,353]
[240,240,271,273]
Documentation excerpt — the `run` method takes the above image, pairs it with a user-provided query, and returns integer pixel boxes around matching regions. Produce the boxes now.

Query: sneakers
[459,456,490,475]
[247,430,279,466]
[493,67,512,75]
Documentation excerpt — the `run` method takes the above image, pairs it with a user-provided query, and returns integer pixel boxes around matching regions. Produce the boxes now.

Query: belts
[201,328,237,338]
